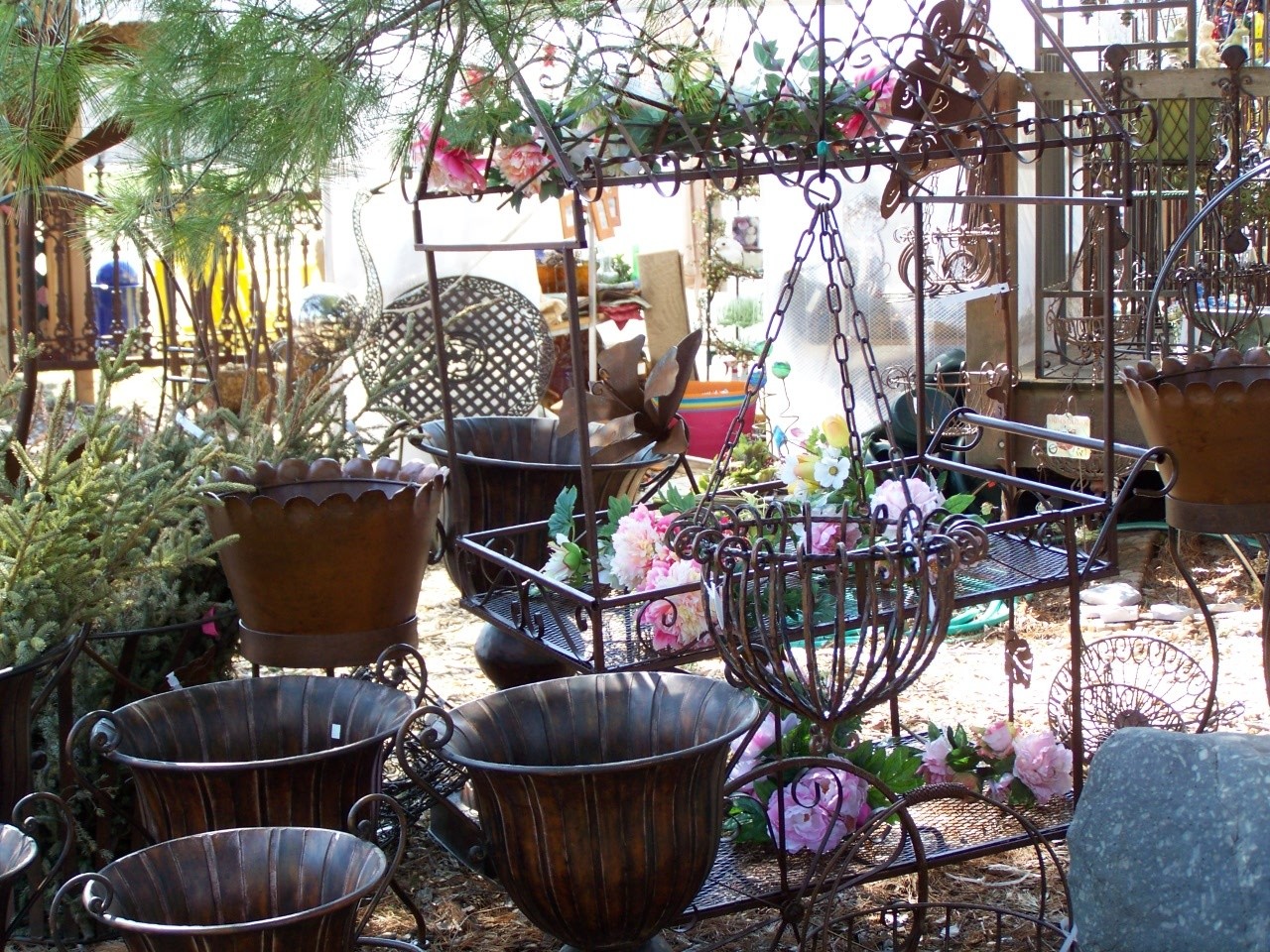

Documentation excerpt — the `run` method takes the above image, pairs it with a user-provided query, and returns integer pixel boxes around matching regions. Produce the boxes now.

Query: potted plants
[0,297,497,823]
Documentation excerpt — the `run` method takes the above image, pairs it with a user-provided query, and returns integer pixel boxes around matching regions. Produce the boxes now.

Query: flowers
[411,58,896,199]
[734,686,1076,858]
[548,426,979,607]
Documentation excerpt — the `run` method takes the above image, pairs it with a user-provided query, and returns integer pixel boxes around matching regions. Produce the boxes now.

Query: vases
[407,415,667,593]
[0,822,38,952]
[432,671,760,951]
[197,455,451,667]
[99,673,415,843]
[84,828,387,952]
[1119,340,1270,533]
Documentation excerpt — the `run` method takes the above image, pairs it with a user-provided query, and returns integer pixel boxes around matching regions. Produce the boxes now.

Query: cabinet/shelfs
[416,1,1181,952]
